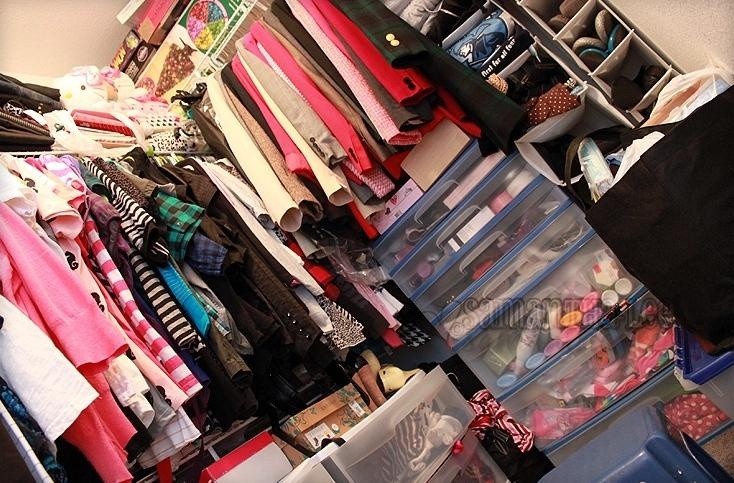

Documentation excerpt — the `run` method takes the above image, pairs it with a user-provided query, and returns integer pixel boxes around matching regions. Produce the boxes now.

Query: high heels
[337,350,420,411]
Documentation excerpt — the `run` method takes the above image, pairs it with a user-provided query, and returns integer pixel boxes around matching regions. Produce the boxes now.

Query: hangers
[197,0,273,76]
[16,149,220,172]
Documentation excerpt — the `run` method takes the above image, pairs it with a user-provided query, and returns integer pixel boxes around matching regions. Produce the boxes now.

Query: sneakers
[549,0,666,110]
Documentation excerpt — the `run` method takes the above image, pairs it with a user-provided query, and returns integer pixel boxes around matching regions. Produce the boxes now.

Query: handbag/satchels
[584,81,733,356]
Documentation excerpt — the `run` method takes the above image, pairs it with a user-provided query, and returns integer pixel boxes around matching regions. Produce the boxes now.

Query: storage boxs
[109,0,192,86]
